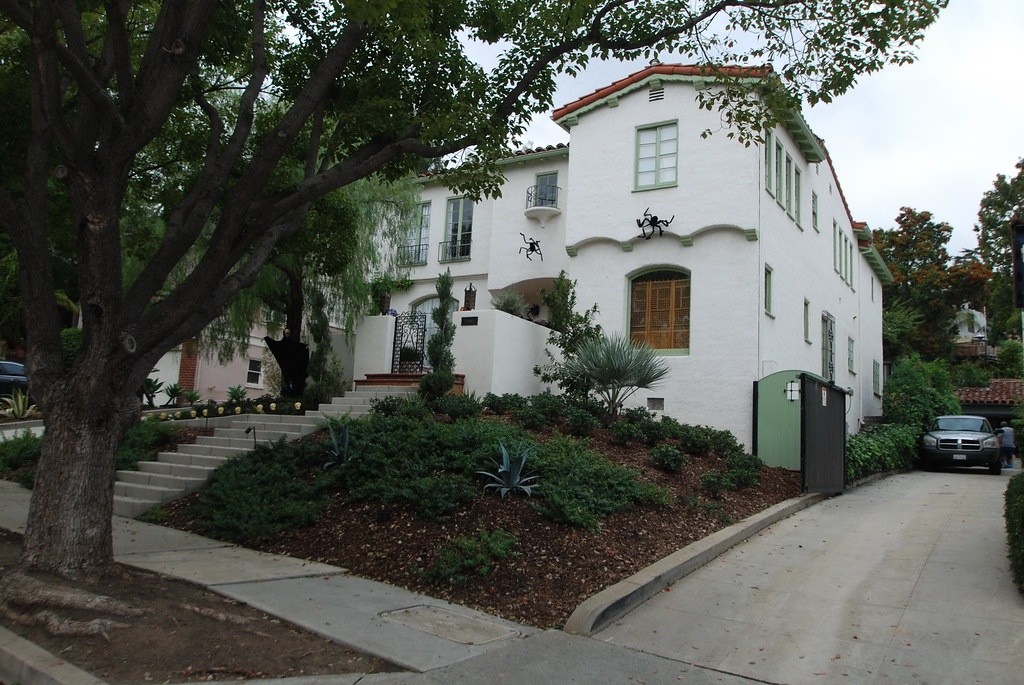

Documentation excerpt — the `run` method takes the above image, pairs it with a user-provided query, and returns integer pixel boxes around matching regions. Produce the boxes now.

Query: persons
[998,421,1015,468]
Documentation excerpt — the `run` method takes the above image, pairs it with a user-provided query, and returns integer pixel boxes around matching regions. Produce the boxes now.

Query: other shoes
[1004,464,1013,468]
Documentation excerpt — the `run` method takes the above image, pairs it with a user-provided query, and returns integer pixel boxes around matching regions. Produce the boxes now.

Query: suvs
[918,414,1005,474]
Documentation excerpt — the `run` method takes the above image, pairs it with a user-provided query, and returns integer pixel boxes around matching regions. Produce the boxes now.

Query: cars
[0,360,30,396]
